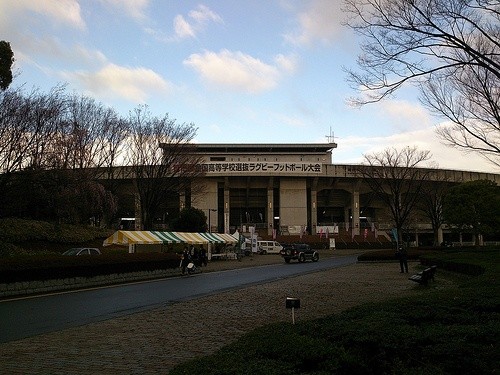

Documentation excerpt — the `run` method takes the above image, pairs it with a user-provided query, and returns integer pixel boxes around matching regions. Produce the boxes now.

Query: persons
[410,238,417,247]
[180,244,207,278]
[398,246,409,274]
[419,240,425,247]
[441,239,456,247]
[401,241,410,254]
[215,243,228,260]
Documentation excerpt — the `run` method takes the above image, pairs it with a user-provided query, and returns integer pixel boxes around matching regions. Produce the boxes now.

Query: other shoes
[400,272,402,273]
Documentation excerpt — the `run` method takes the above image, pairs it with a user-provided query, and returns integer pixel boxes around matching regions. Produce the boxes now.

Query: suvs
[280,243,320,264]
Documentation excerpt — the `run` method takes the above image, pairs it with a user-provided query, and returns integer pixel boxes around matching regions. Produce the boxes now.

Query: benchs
[408,266,437,286]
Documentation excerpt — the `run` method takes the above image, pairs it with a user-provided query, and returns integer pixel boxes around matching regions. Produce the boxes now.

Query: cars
[60,247,103,257]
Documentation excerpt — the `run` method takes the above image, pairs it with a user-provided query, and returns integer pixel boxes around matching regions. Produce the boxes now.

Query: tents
[102,231,212,262]
[200,232,239,261]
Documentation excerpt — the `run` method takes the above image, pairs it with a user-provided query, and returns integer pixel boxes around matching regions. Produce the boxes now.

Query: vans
[256,240,286,255]
